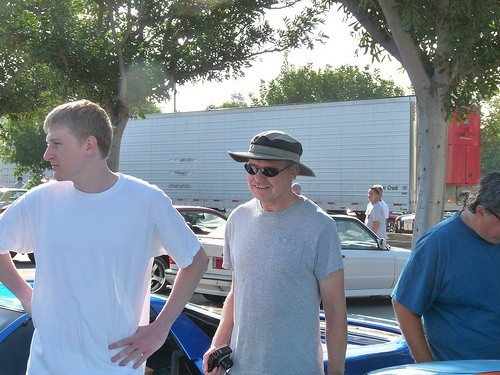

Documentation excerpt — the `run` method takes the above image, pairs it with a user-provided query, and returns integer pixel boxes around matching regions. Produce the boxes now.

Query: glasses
[244,159,294,177]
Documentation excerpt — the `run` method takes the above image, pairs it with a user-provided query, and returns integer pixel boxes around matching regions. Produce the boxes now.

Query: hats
[227,131,316,177]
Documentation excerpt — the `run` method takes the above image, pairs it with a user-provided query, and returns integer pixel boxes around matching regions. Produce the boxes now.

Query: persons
[292,183,301,195]
[0,100,208,375]
[14,177,24,189]
[390,173,500,364]
[202,130,348,375]
[364,185,389,244]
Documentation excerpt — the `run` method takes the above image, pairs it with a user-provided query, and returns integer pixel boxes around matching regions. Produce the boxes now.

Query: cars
[0,281,500,375]
[0,188,38,264]
[151,205,460,303]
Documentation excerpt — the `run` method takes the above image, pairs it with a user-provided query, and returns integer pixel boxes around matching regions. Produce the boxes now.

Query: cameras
[207,345,233,373]
